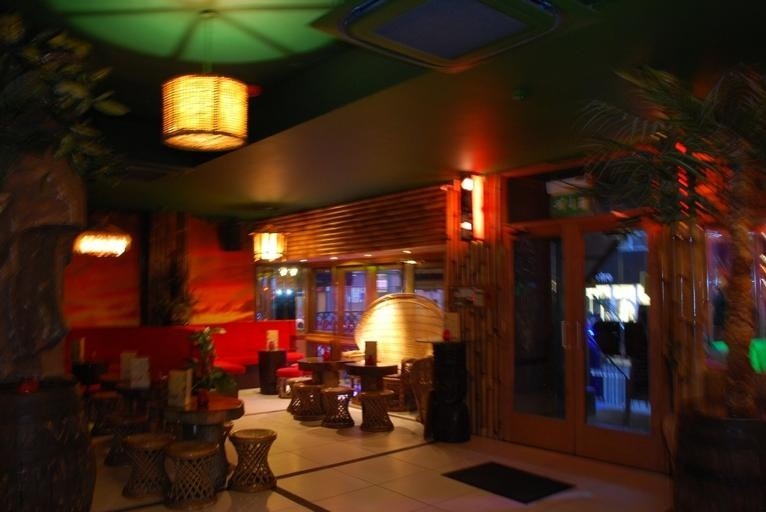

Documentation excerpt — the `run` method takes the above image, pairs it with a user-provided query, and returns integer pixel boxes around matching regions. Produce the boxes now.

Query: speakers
[258,350,285,395]
[220,222,243,251]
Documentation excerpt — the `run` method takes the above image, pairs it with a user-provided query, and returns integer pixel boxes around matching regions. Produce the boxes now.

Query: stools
[275,351,312,412]
[165,442,220,511]
[77,376,168,467]
[358,389,394,432]
[294,384,325,421]
[121,432,176,499]
[227,428,278,493]
[320,387,355,428]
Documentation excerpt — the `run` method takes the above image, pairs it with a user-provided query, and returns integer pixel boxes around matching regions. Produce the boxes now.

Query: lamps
[162,8,252,150]
[247,207,289,263]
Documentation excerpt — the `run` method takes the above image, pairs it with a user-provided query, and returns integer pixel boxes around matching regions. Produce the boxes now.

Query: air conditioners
[303,0,608,80]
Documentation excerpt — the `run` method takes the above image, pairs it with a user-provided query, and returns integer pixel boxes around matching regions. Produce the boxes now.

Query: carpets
[443,459,575,506]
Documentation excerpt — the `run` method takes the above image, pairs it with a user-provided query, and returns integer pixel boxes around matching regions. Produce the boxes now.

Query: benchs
[339,292,448,411]
[67,321,298,389]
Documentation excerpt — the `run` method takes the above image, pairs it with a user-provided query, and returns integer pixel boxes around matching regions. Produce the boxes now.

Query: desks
[297,357,356,388]
[150,393,244,490]
[410,335,479,445]
[345,360,398,393]
